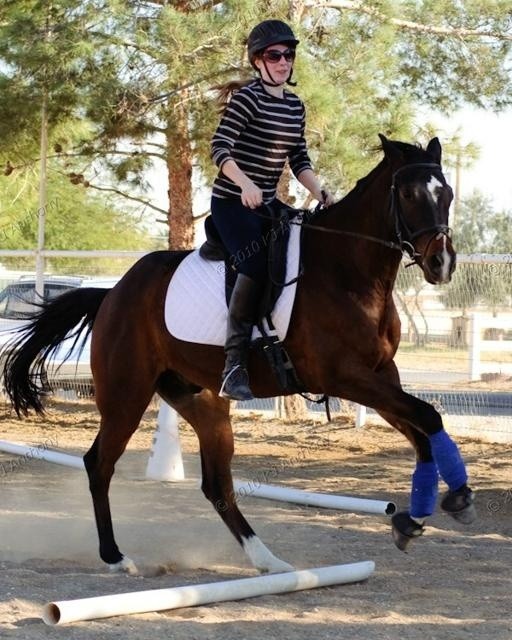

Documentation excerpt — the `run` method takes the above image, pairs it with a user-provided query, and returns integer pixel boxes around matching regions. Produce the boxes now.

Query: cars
[1,271,120,398]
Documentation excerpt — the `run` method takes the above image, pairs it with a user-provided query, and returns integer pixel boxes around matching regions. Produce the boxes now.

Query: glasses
[264,48,296,63]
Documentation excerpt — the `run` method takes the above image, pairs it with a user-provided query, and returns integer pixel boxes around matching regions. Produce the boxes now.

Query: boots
[222,273,261,400]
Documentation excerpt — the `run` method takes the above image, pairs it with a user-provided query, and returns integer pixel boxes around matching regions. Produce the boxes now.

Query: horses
[0,129,481,577]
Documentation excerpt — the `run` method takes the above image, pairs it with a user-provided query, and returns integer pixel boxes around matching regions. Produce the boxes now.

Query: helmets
[246,19,300,72]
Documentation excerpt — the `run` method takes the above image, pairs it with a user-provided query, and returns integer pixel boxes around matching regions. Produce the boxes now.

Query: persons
[209,20,333,402]
